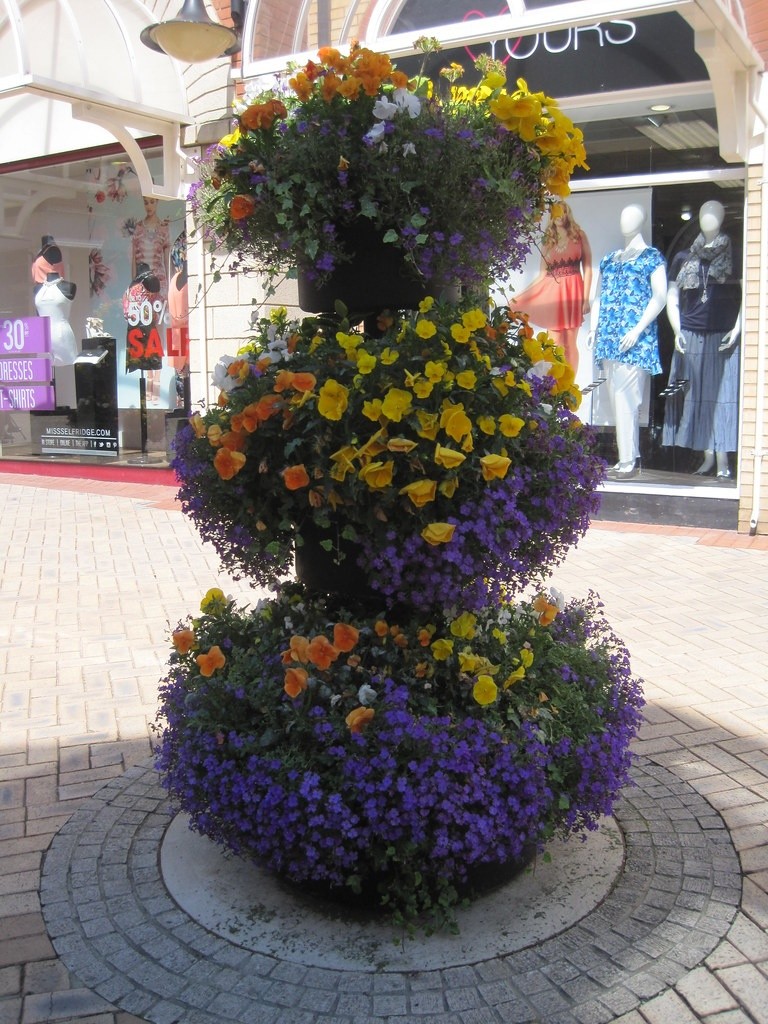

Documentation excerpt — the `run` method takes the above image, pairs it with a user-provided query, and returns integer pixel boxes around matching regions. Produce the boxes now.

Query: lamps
[139,0,241,64]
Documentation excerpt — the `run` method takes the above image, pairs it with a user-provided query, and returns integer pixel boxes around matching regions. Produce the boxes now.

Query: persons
[32,235,78,365]
[132,195,170,405]
[168,227,190,375]
[507,201,592,386]
[587,200,743,480]
[122,263,163,372]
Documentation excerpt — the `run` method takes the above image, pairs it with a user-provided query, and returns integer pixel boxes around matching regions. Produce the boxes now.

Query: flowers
[146,582,646,940]
[164,304,607,614]
[169,34,589,310]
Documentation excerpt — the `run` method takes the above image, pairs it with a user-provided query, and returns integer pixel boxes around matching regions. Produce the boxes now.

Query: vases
[301,846,535,909]
[297,215,461,315]
[294,513,369,597]
